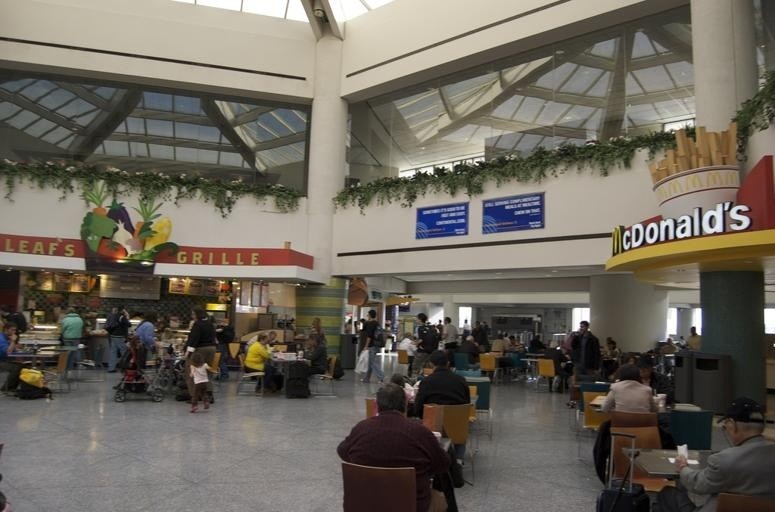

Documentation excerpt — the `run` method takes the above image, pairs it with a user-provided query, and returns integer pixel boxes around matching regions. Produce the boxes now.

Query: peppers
[86,232,101,253]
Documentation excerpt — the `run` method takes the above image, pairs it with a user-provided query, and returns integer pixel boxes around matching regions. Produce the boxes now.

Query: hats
[714,396,765,423]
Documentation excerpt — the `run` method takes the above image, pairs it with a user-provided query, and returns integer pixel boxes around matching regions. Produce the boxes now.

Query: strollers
[113,332,189,403]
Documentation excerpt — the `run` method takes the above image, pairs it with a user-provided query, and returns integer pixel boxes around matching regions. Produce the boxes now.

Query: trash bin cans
[673,352,734,414]
[339,334,360,369]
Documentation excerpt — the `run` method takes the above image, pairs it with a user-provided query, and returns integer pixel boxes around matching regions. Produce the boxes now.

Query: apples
[93,208,108,216]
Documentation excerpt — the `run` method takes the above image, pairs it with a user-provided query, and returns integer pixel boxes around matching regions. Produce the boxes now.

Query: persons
[344,310,547,383]
[0,322,25,397]
[181,305,330,413]
[336,382,451,511]
[0,303,18,348]
[564,321,703,450]
[414,350,470,488]
[375,373,415,415]
[656,397,775,512]
[103,304,160,394]
[60,306,84,371]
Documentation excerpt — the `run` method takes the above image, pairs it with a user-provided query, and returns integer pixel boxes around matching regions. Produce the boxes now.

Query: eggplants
[106,200,135,234]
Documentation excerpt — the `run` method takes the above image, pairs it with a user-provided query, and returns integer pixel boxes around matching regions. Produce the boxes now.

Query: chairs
[46,350,71,393]
[0,370,9,392]
[210,353,221,381]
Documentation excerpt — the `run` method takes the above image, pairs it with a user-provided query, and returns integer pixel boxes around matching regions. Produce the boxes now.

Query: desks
[8,325,61,390]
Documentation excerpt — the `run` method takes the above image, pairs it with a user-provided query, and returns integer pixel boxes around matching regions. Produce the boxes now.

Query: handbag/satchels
[374,329,384,347]
[103,313,119,331]
[423,326,439,353]
[17,368,45,398]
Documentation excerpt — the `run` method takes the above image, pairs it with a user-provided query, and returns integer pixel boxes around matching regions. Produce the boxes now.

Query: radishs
[112,219,134,249]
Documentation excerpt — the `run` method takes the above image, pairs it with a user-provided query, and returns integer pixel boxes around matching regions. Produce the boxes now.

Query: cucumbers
[152,243,179,255]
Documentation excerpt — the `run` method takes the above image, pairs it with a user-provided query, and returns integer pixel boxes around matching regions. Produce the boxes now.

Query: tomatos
[98,238,128,259]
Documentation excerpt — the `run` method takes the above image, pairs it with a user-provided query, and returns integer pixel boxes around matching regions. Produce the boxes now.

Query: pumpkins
[145,217,172,249]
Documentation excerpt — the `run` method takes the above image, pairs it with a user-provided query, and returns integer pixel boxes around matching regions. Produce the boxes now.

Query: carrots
[126,222,146,252]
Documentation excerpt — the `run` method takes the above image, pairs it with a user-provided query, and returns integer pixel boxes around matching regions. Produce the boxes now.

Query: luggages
[327,357,345,378]
[285,360,311,398]
[595,432,650,511]
